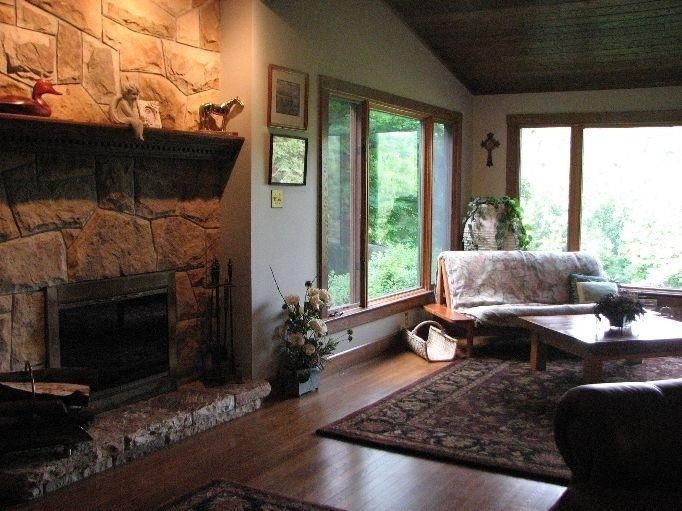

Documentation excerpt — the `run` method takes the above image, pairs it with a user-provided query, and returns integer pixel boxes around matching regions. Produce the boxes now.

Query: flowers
[267,264,356,378]
[591,291,649,328]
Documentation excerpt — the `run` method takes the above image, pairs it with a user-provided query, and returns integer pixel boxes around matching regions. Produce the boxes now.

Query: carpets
[144,474,344,509]
[315,357,681,489]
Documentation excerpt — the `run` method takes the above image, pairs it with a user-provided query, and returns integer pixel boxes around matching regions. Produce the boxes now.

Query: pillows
[567,272,623,308]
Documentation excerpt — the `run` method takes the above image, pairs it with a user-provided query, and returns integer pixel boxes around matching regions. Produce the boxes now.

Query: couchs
[538,373,681,511]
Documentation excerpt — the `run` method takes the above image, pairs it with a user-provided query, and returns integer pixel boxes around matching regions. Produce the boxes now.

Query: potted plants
[462,192,534,253]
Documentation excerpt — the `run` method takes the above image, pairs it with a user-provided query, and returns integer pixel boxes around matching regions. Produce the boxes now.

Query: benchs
[425,246,630,364]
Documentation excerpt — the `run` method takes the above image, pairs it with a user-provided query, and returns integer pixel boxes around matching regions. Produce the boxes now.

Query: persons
[109,84,146,142]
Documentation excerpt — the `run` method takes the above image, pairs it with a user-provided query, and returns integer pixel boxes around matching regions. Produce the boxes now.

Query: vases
[603,311,633,329]
[272,364,324,400]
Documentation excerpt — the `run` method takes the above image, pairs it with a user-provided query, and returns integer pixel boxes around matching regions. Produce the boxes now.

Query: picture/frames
[268,131,310,190]
[266,61,311,133]
